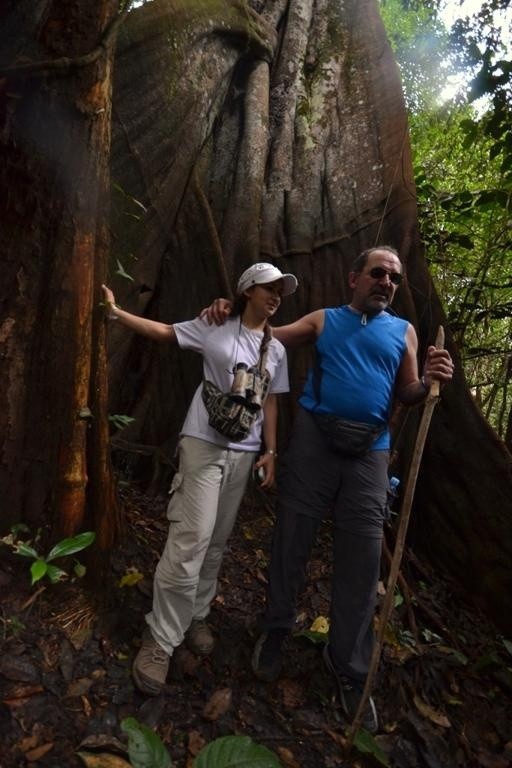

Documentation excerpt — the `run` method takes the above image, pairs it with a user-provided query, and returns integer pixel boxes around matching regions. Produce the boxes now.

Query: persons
[102,262,299,696]
[198,245,455,729]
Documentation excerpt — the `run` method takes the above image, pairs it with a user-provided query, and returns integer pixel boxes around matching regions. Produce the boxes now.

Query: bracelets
[421,376,429,391]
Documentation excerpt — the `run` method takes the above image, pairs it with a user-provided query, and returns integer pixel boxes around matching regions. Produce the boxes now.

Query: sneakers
[250,629,283,682]
[323,642,378,733]
[133,627,170,697]
[185,620,214,656]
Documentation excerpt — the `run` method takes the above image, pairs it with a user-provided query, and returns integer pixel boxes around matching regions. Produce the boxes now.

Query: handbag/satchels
[202,380,261,442]
[312,412,387,457]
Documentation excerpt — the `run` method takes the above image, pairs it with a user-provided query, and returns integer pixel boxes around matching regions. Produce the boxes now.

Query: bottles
[388,476,400,508]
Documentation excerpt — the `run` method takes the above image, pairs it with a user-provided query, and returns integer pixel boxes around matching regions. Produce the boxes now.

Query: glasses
[370,267,402,284]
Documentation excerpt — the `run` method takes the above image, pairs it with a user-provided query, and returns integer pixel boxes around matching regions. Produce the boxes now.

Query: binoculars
[230,363,263,411]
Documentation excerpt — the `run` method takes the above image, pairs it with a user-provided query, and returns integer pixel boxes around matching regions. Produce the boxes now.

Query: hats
[236,263,299,297]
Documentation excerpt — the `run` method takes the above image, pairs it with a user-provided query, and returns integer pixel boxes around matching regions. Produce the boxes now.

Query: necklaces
[350,304,379,326]
